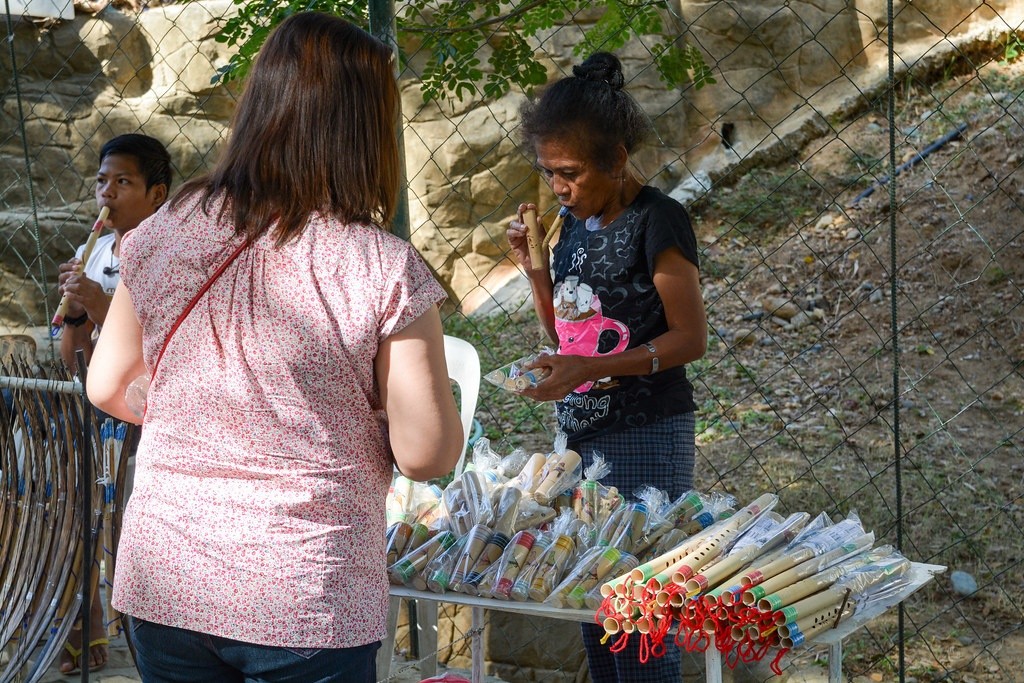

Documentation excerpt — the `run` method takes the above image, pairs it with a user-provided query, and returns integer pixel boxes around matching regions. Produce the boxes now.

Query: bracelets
[63,311,88,327]
[642,342,660,375]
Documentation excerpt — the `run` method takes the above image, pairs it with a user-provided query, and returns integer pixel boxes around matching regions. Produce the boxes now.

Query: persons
[506,51,707,683]
[86,11,465,683]
[0,133,176,674]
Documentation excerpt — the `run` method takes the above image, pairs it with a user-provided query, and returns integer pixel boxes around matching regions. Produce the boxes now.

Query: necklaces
[103,251,119,275]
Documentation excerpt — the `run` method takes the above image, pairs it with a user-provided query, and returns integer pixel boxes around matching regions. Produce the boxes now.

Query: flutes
[48,205,112,328]
[590,493,912,650]
